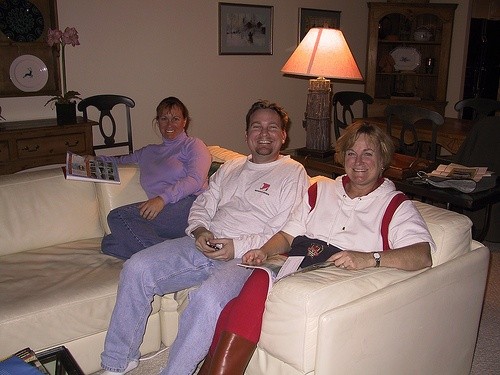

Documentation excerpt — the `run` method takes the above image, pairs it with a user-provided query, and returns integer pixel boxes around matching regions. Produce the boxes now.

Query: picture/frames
[218,2,274,56]
[297,7,342,45]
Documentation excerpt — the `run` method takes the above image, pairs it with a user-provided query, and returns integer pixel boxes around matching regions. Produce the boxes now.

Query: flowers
[44,27,87,109]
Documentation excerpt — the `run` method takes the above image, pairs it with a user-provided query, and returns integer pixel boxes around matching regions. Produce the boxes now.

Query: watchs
[372,251,381,268]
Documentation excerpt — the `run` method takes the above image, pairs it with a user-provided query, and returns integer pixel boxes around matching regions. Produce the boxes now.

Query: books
[66,151,121,184]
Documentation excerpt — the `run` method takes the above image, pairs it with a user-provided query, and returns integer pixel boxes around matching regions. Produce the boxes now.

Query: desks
[281,148,434,199]
[405,170,500,245]
[353,118,475,155]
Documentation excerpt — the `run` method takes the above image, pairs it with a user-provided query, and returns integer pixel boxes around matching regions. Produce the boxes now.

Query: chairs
[78,95,136,157]
[332,91,500,243]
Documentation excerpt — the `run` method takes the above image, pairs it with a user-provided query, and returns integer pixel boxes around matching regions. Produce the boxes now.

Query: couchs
[0,146,489,375]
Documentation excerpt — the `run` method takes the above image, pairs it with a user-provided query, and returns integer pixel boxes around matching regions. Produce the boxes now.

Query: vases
[56,102,77,125]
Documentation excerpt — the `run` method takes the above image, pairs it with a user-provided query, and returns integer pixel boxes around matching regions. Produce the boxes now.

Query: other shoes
[101,360,140,375]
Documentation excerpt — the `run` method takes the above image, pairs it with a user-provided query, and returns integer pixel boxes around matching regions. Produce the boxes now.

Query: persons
[197,120,437,375]
[101,99,310,375]
[82,96,211,259]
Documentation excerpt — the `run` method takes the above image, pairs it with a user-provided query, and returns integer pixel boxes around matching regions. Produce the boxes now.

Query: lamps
[280,28,366,159]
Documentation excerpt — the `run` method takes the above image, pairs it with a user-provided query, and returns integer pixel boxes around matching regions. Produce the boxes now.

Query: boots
[197,332,257,375]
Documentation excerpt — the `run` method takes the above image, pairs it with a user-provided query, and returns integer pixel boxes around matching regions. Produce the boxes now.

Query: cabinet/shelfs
[367,2,460,159]
[0,116,100,175]
